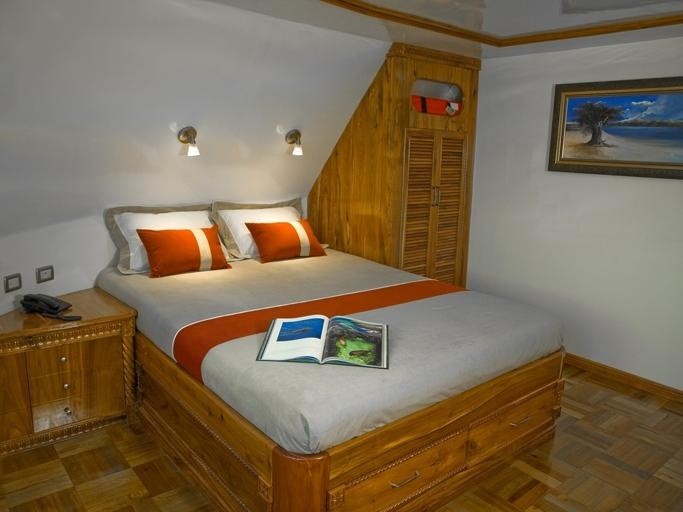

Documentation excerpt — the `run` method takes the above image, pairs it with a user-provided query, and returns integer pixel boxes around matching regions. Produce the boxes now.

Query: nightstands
[0,286,139,461]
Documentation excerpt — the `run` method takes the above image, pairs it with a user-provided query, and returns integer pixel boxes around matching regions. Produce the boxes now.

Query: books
[256,314,389,370]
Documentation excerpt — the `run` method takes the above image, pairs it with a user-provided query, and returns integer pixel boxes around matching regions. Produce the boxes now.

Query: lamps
[274,121,305,158]
[166,118,203,159]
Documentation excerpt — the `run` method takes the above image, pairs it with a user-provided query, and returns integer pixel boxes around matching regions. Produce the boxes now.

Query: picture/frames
[546,77,682,180]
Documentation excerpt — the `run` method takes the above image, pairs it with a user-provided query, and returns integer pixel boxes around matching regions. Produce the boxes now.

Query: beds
[94,195,568,512]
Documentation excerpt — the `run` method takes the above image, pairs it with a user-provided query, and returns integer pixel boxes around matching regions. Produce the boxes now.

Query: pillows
[135,224,233,278]
[205,197,329,261]
[243,219,327,264]
[103,202,248,276]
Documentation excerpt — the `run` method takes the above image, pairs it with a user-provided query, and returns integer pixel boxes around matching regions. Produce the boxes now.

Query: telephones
[20,293,72,314]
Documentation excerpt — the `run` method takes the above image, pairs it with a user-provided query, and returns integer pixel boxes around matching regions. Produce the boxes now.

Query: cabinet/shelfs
[308,42,482,289]
